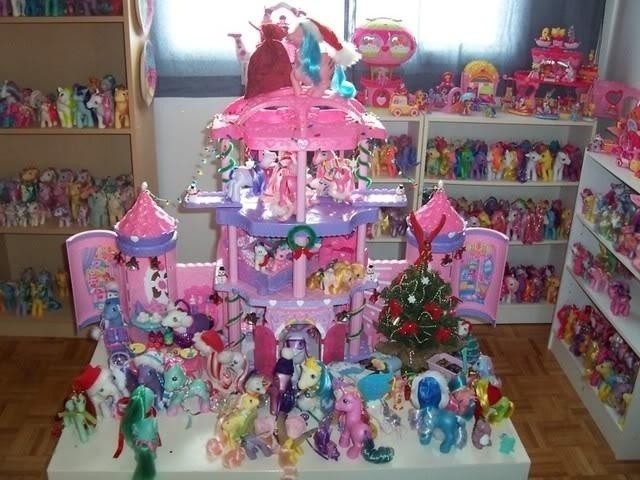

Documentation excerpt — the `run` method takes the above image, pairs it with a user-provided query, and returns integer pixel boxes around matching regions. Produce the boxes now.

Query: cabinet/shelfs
[418,98,601,324]
[0,1,160,340]
[347,114,423,260]
[543,143,639,462]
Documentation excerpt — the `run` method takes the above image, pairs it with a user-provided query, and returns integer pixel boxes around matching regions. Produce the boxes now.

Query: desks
[47,331,532,480]
